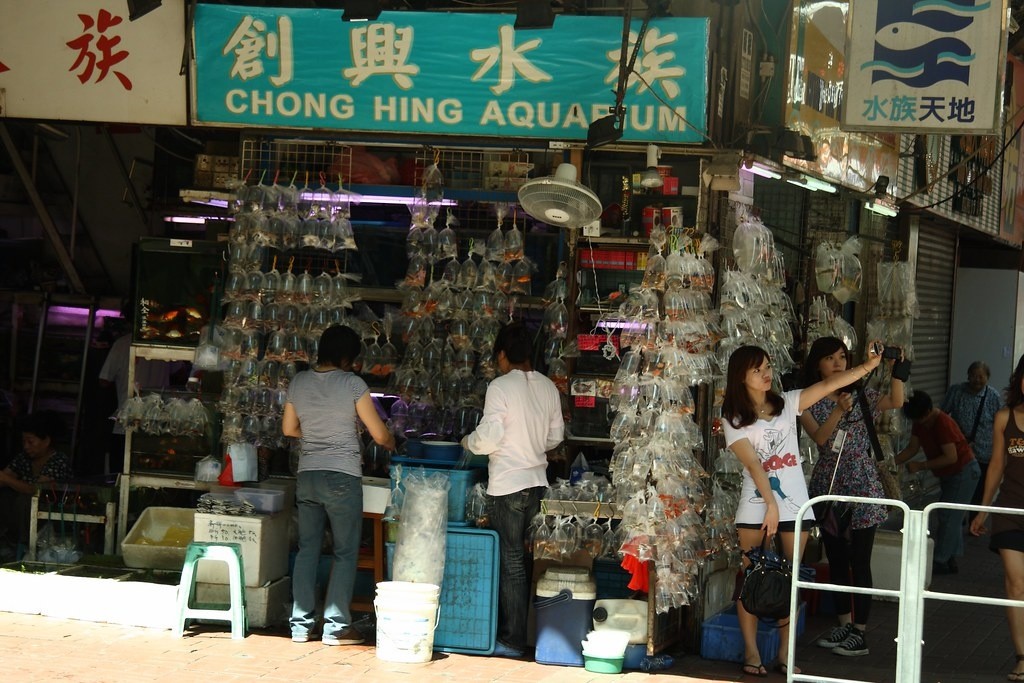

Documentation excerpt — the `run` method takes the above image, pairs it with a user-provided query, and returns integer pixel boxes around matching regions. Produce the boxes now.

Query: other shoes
[323,623,364,645]
[291,631,309,642]
[933,557,958,576]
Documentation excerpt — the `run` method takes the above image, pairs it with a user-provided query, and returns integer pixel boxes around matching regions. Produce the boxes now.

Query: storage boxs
[700,528,934,666]
[133,234,227,350]
[0,458,678,666]
[135,387,222,481]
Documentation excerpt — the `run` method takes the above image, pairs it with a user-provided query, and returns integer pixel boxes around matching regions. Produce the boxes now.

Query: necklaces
[754,402,767,414]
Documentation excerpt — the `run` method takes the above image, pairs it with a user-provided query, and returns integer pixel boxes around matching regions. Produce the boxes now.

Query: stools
[174,541,251,641]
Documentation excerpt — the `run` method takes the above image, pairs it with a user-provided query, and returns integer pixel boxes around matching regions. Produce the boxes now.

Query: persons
[894,389,982,592]
[719,340,885,677]
[280,326,396,645]
[459,324,566,657]
[969,355,1024,682]
[941,360,1004,533]
[798,335,907,659]
[98,321,187,477]
[0,409,81,552]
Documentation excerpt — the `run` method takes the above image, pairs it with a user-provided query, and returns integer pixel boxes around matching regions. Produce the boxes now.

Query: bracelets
[862,364,871,373]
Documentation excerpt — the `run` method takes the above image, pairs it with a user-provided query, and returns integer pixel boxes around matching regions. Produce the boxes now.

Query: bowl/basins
[420,439,460,462]
[581,652,624,674]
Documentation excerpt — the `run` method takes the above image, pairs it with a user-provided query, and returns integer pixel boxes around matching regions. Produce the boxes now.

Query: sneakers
[832,629,870,655]
[816,623,853,647]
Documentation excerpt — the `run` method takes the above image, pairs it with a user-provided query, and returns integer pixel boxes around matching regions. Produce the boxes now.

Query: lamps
[163,192,456,222]
[513,0,556,29]
[741,154,786,180]
[582,0,659,150]
[785,172,838,193]
[753,129,817,162]
[864,198,900,217]
[872,177,889,199]
[703,152,742,192]
[642,144,664,187]
[127,0,162,21]
[341,0,383,21]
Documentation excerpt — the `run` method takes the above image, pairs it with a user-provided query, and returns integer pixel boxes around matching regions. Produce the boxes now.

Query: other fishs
[115,188,860,609]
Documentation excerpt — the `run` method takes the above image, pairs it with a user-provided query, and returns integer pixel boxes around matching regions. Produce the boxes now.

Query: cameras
[870,342,880,356]
[882,347,901,359]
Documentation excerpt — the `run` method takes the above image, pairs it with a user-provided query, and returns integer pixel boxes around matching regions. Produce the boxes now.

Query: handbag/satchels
[872,432,901,510]
[737,523,816,628]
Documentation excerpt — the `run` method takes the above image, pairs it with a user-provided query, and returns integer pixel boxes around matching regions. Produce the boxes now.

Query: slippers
[1007,657,1024,683]
[775,662,801,676]
[742,658,766,675]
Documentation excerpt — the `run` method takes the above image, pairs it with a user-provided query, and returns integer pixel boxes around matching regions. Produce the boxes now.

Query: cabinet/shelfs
[280,227,652,444]
[116,344,222,555]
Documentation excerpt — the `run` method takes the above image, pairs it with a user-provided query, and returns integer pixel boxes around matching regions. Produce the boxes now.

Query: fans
[518,163,603,228]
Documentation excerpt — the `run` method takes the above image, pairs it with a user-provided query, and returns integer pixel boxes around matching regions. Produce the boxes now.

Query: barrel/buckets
[372,581,441,663]
